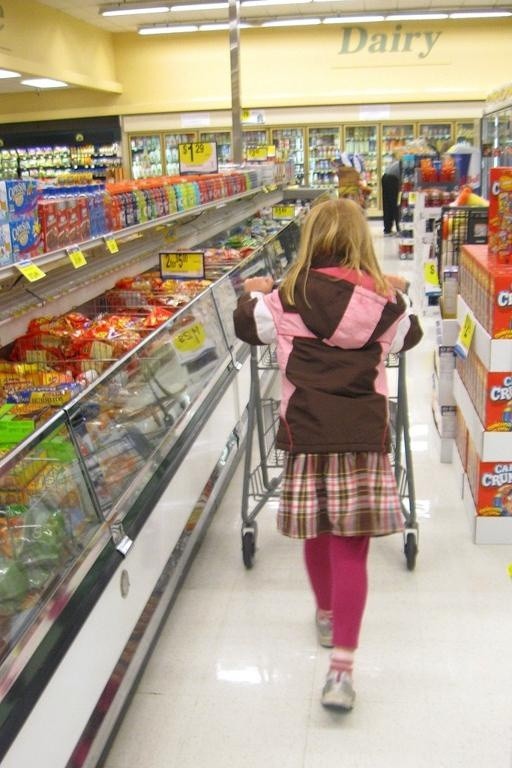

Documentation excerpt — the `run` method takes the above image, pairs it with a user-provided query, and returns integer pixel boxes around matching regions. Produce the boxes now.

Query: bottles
[310,124,474,185]
[56,128,304,229]
[442,208,467,268]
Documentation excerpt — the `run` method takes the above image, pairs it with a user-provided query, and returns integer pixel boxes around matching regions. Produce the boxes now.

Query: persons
[233,197,423,711]
[381,161,403,237]
[331,159,373,209]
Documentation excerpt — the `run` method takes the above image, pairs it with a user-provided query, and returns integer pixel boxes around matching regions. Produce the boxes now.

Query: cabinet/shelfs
[269,122,343,185]
[127,128,198,176]
[343,119,417,217]
[0,178,332,767]
[417,118,480,156]
[198,125,269,166]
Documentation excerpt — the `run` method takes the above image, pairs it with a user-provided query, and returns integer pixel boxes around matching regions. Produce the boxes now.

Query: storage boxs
[427,166,512,546]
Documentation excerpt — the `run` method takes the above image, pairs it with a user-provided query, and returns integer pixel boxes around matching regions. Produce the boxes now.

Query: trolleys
[241,279,420,570]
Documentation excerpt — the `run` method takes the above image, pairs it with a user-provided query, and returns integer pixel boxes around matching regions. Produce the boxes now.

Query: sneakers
[315,611,333,649]
[321,671,355,710]
